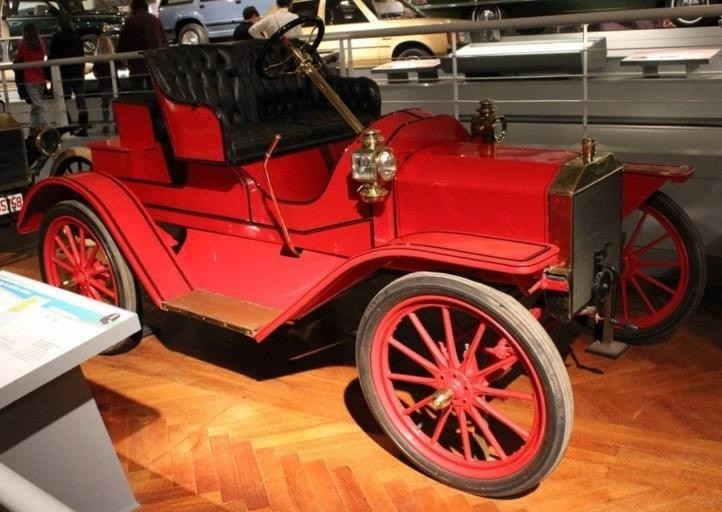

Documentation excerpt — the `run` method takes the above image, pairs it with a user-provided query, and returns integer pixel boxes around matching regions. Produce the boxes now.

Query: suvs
[0,96,98,260]
[8,10,713,503]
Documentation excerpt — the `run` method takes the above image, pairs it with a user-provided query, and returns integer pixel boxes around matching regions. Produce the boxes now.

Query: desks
[0,267,145,512]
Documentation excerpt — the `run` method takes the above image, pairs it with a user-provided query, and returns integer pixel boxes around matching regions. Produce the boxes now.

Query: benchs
[144,33,382,169]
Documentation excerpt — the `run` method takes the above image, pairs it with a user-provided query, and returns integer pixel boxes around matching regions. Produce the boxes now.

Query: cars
[155,0,278,48]
[419,0,720,40]
[0,0,128,63]
[283,0,474,67]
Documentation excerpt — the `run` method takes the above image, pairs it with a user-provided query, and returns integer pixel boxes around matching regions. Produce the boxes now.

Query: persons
[231,6,259,41]
[117,0,168,92]
[93,34,127,133]
[45,13,88,131]
[248,0,302,43]
[16,21,47,136]
